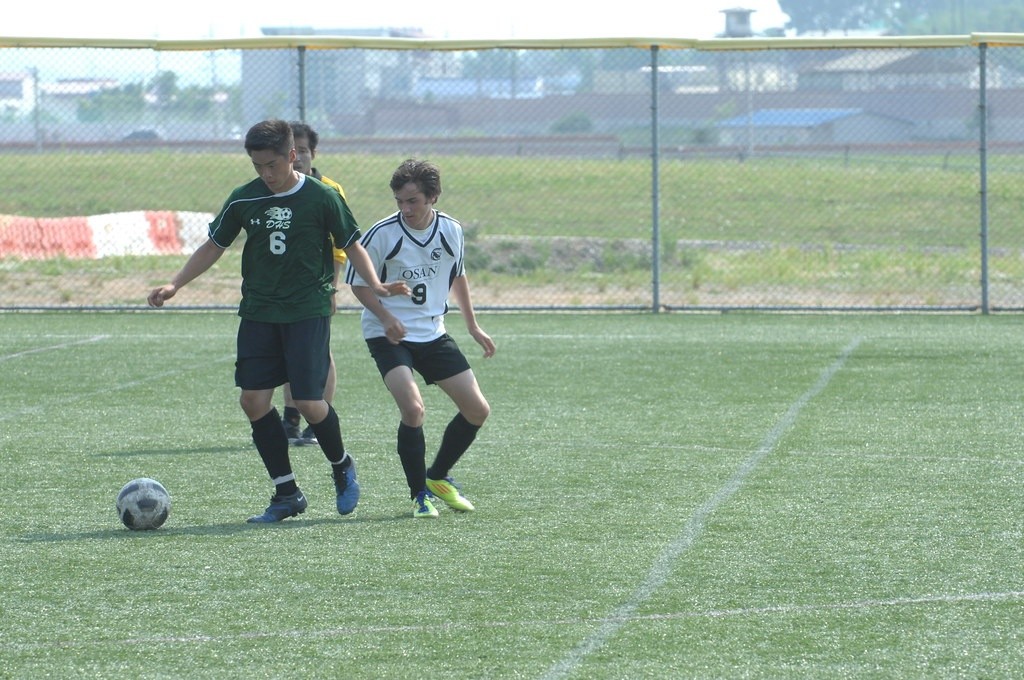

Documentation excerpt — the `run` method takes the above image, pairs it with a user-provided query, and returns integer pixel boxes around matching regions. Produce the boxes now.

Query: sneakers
[247,487,307,523]
[282,419,304,446]
[300,425,318,444]
[411,490,439,518]
[426,468,475,512]
[331,453,360,515]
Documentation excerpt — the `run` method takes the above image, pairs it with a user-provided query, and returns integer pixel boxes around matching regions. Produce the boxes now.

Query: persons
[148,119,409,525]
[344,159,497,520]
[281,123,345,448]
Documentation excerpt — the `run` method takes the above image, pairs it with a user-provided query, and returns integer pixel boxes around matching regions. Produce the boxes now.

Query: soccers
[115,476,173,530]
